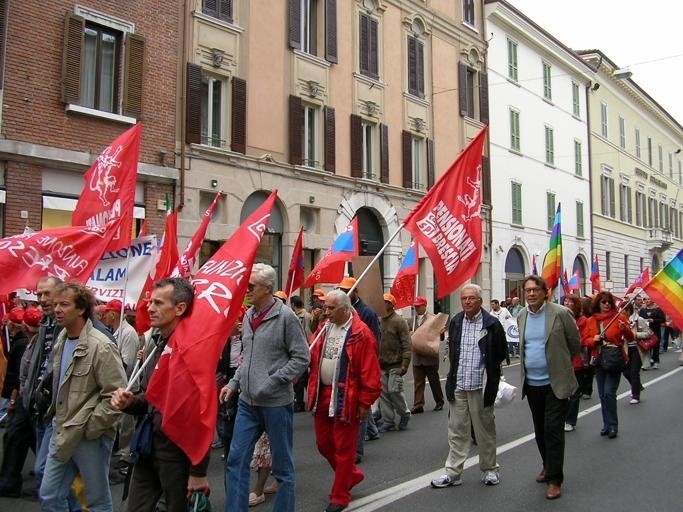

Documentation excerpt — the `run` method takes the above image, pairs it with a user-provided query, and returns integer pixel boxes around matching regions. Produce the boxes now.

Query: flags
[3,121,682,465]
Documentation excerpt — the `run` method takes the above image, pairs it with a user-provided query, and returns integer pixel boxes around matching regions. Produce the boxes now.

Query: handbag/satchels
[637,334,659,352]
[598,344,626,372]
[129,418,153,456]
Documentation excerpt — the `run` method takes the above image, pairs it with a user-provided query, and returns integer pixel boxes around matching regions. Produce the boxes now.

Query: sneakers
[249,491,265,506]
[211,440,224,448]
[630,399,638,404]
[431,475,463,488]
[481,470,499,484]
[564,423,616,438]
[264,485,279,494]
[109,461,129,481]
[365,404,445,441]
[583,394,590,399]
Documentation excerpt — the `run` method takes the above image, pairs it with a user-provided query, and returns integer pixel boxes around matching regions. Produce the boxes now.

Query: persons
[2,265,682,512]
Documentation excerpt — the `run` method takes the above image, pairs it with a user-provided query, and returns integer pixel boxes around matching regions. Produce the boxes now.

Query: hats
[273,291,287,300]
[414,297,426,305]
[6,306,24,323]
[98,300,122,312]
[384,293,396,305]
[23,308,42,326]
[312,277,357,301]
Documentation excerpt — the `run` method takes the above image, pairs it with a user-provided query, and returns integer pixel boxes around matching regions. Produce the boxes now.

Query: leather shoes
[536,469,545,481]
[326,503,343,512]
[24,489,37,496]
[547,483,560,498]
